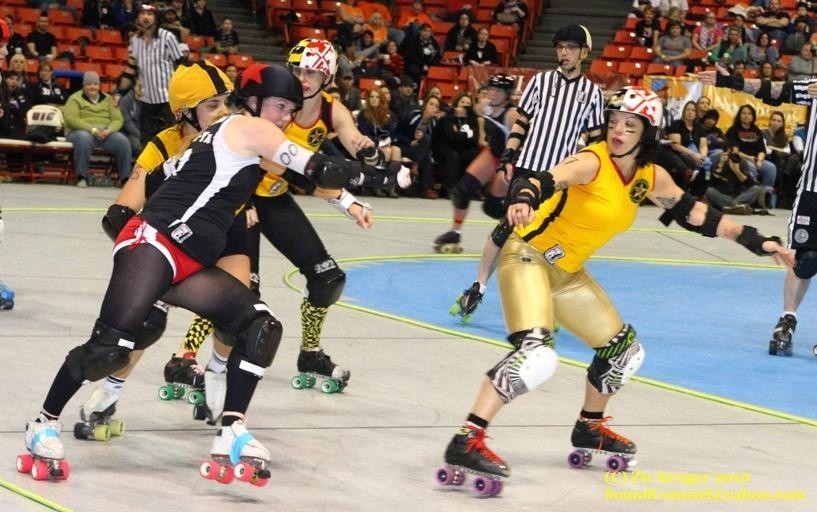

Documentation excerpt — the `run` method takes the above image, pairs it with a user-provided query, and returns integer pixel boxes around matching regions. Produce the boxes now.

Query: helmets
[232,61,305,113]
[551,23,593,52]
[168,58,240,116]
[135,2,158,14]
[486,72,516,92]
[601,85,665,128]
[283,35,339,80]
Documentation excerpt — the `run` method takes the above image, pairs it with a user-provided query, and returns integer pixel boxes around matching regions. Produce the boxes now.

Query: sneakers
[77,178,87,188]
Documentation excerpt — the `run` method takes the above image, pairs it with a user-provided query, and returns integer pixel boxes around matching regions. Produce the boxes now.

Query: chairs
[588,16,685,80]
[419,0,542,107]
[263,0,337,48]
[689,1,815,81]
[0,0,130,97]
[360,78,386,100]
[181,34,253,70]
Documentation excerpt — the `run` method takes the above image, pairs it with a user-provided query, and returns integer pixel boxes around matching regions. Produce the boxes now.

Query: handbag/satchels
[28,125,57,144]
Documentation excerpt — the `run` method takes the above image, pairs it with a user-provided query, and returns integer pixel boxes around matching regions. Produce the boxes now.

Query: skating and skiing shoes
[0,281,15,309]
[291,349,352,396]
[15,420,71,481]
[450,283,487,323]
[193,369,229,426]
[74,390,126,441]
[567,418,638,475]
[435,429,512,498]
[433,230,465,255]
[159,355,207,406]
[200,420,274,490]
[767,315,800,356]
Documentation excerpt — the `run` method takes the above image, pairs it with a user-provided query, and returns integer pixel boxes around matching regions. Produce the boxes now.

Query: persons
[75,59,373,442]
[629,1,815,217]
[14,62,412,487]
[696,62,817,358]
[435,84,796,500]
[158,35,381,405]
[1,0,524,199]
[427,68,525,256]
[434,23,607,325]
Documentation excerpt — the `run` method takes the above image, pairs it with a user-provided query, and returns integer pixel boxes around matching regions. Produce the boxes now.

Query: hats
[400,77,415,88]
[341,70,354,79]
[83,71,101,86]
[4,70,20,79]
